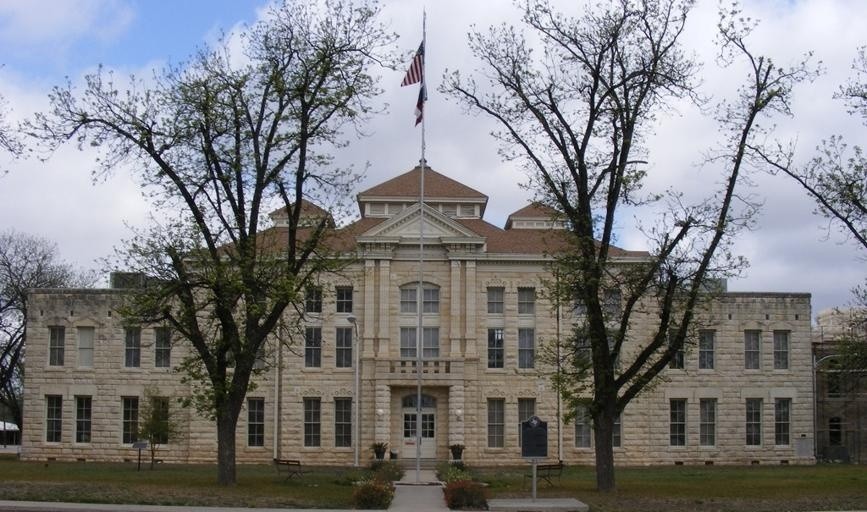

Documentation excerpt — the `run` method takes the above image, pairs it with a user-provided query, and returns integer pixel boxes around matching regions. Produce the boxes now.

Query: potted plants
[370,443,389,459]
[448,445,464,459]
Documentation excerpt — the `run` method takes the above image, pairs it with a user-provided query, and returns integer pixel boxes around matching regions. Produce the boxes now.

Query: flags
[413,78,427,128]
[400,38,424,88]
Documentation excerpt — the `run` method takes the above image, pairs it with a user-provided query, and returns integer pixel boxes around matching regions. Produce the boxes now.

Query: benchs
[523,465,564,490]
[274,458,314,482]
